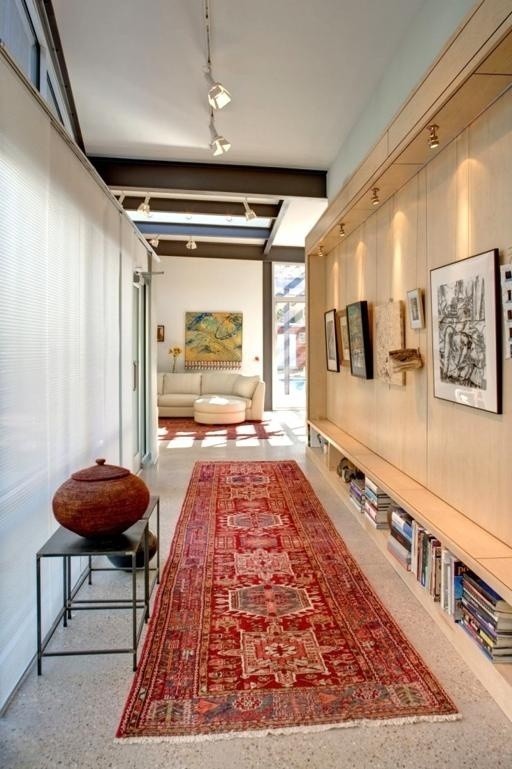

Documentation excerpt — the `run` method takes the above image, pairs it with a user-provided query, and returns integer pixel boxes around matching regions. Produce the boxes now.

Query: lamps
[370,186,381,208]
[317,241,324,257]
[136,191,153,218]
[242,199,257,222]
[339,221,347,237]
[427,122,442,150]
[203,0,233,112]
[204,106,232,156]
[149,232,161,248]
[185,232,197,250]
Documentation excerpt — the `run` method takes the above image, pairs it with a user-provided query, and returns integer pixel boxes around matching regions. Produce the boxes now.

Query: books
[348,477,512,664]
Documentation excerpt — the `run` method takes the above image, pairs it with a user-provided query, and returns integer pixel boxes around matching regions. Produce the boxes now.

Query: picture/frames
[345,298,373,381]
[156,325,165,343]
[323,310,341,373]
[407,287,425,330]
[428,249,503,418]
[336,309,351,367]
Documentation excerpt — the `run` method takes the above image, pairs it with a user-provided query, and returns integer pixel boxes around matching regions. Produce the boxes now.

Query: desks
[35,519,149,676]
[67,496,159,619]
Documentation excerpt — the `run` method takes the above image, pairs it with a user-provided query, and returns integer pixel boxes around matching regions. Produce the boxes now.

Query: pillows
[232,374,260,399]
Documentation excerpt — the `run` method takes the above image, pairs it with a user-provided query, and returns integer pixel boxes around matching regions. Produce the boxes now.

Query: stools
[194,398,246,427]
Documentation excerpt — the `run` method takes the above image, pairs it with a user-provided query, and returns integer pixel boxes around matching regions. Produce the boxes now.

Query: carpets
[158,417,270,441]
[110,460,462,747]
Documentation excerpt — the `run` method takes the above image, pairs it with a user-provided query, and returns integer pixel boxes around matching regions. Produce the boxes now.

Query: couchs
[154,370,267,423]
